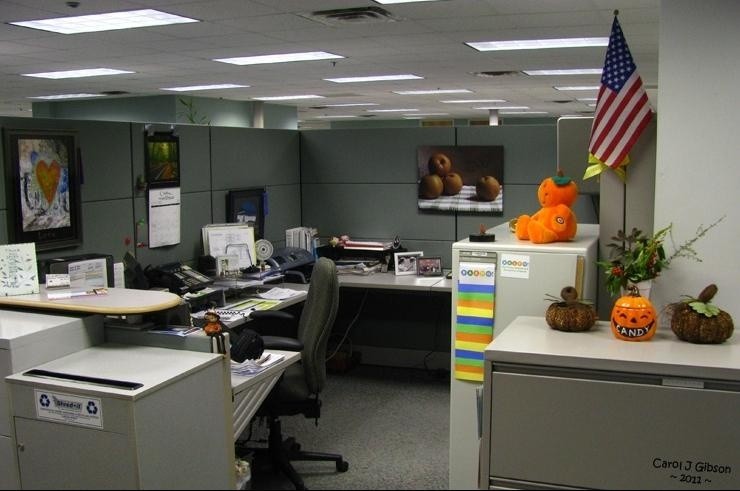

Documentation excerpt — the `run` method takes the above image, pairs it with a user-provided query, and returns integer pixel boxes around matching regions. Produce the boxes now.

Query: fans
[256,239,273,268]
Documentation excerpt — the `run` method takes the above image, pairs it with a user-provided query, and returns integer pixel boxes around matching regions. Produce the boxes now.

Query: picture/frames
[417,257,442,275]
[145,137,180,184]
[2,129,83,252]
[227,188,265,241]
[395,252,423,275]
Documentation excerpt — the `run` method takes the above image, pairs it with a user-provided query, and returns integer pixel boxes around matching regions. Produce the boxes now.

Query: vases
[621,278,652,300]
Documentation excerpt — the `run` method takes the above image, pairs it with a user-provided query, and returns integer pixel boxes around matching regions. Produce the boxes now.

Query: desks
[195,282,342,329]
[336,268,452,292]
[431,279,451,292]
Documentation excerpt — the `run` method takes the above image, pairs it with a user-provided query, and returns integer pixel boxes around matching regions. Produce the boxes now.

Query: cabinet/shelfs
[480,316,739,490]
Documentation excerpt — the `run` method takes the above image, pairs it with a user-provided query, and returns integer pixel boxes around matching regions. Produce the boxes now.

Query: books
[284,228,317,252]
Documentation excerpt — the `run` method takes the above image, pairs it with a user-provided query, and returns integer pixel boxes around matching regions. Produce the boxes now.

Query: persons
[430,264,437,273]
[406,257,416,272]
[398,259,408,272]
[425,264,429,271]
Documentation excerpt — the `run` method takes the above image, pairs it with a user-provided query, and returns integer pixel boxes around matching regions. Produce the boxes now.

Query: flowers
[595,226,671,298]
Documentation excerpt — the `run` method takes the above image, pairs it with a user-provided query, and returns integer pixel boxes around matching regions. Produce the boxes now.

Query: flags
[587,15,654,172]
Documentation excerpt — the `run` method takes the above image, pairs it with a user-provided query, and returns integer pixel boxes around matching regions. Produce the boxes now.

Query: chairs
[235,257,348,490]
